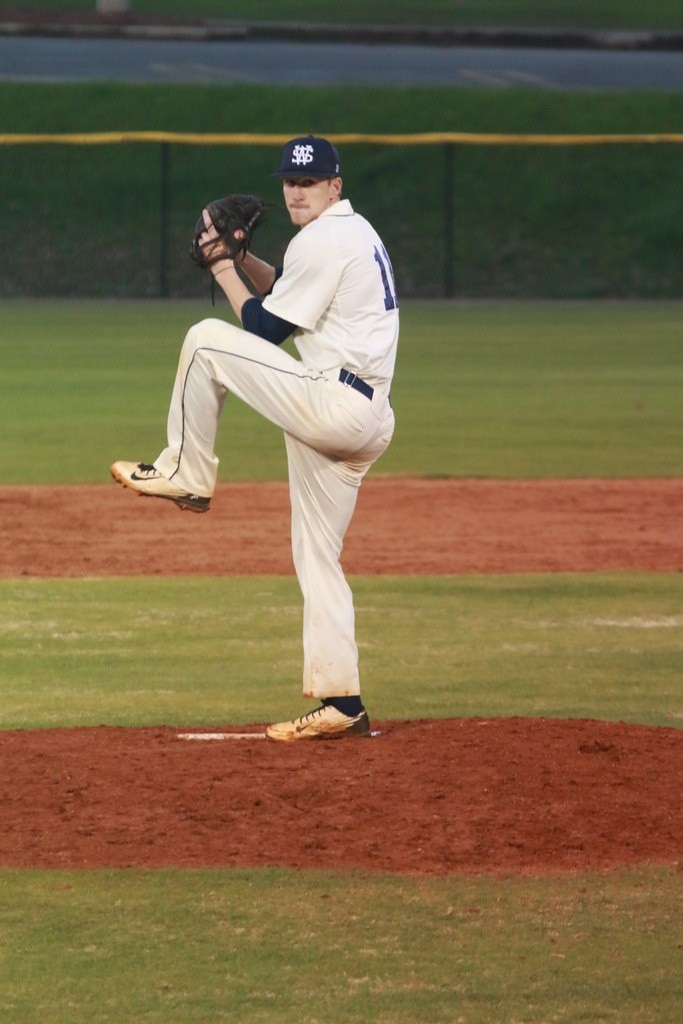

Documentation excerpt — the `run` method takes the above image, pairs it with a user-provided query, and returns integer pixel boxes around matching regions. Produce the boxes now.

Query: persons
[110,133,398,741]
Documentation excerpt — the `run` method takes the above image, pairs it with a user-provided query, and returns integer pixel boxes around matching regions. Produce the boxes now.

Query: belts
[338,368,392,408]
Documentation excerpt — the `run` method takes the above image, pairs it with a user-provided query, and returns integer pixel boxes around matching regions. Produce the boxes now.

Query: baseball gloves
[189,196,264,268]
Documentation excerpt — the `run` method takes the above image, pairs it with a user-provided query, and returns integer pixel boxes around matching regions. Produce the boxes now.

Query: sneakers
[263,704,372,744]
[109,459,210,515]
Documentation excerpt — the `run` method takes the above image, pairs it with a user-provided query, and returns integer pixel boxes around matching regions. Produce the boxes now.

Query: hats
[271,135,340,178]
